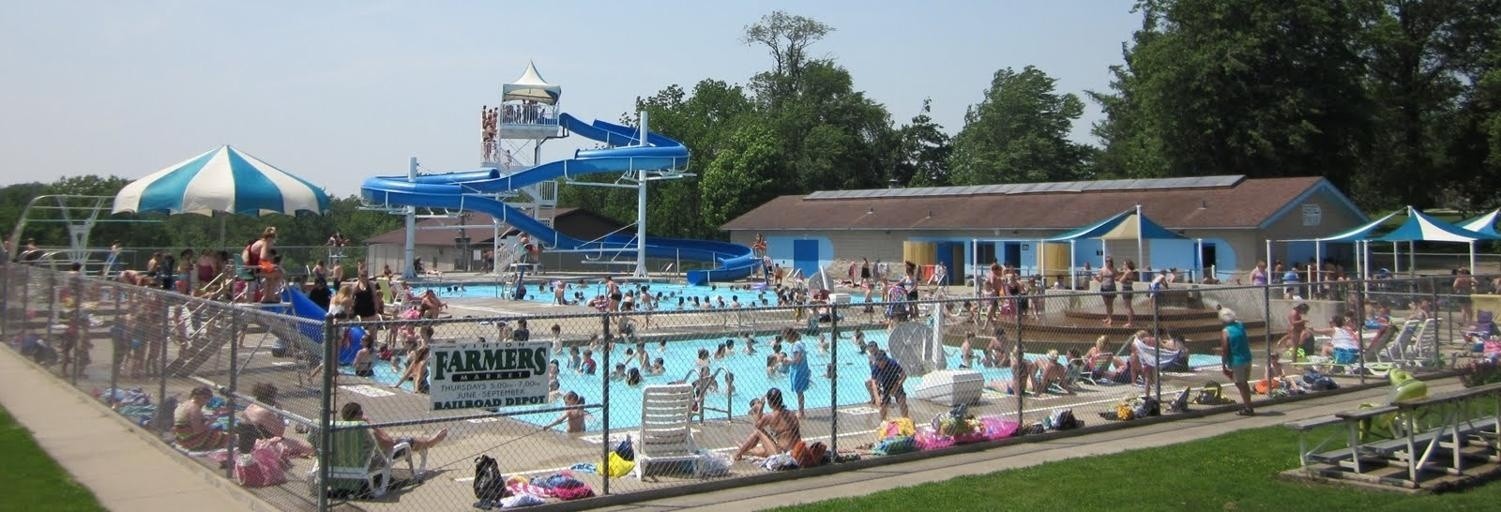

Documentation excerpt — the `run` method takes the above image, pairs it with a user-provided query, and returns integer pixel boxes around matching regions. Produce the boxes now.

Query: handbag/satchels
[235,454,259,486]
[474,455,505,498]
[1199,380,1222,403]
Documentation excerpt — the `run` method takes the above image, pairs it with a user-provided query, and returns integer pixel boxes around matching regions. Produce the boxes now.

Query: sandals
[1236,407,1253,415]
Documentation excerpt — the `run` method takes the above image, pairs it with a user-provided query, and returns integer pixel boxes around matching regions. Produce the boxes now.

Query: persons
[0,229,1501,475]
[478,94,549,168]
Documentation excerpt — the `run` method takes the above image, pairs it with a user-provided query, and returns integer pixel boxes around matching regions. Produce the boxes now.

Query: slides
[280,284,365,365]
[361,113,762,288]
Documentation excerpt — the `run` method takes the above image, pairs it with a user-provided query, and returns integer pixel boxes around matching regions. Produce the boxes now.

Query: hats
[1219,309,1235,323]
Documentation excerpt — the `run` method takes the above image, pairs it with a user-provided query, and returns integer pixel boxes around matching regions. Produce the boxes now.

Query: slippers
[473,499,502,510]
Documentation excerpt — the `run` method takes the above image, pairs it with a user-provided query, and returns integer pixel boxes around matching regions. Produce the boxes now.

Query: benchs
[1283,382,1501,493]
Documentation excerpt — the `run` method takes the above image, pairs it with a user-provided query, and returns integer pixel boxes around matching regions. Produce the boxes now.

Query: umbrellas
[111,143,335,239]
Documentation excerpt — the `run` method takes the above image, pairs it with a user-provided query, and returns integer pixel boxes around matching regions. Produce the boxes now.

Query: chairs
[630,384,702,481]
[1274,317,1442,378]
[309,421,426,495]
[1080,353,1114,387]
[1052,359,1084,393]
